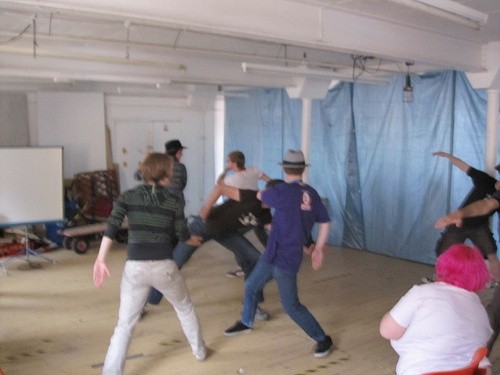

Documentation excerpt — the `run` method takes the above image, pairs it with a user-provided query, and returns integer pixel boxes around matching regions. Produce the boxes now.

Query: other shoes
[226,266,245,277]
[253,305,269,320]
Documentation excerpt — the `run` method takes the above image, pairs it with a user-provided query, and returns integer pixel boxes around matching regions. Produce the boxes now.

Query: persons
[379,243,494,375]
[216,151,272,277]
[92,151,210,375]
[223,149,333,356]
[132,140,188,209]
[422,151,500,289]
[139,179,317,320]
[434,189,500,228]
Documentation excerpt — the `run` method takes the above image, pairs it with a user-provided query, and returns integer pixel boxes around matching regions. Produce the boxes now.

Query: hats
[166,140,186,152]
[279,149,311,169]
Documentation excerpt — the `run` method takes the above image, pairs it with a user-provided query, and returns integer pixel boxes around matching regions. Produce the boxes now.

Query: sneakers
[225,319,252,337]
[313,335,333,358]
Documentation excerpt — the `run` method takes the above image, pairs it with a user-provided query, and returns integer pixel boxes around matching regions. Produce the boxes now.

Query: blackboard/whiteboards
[0,146,63,224]
